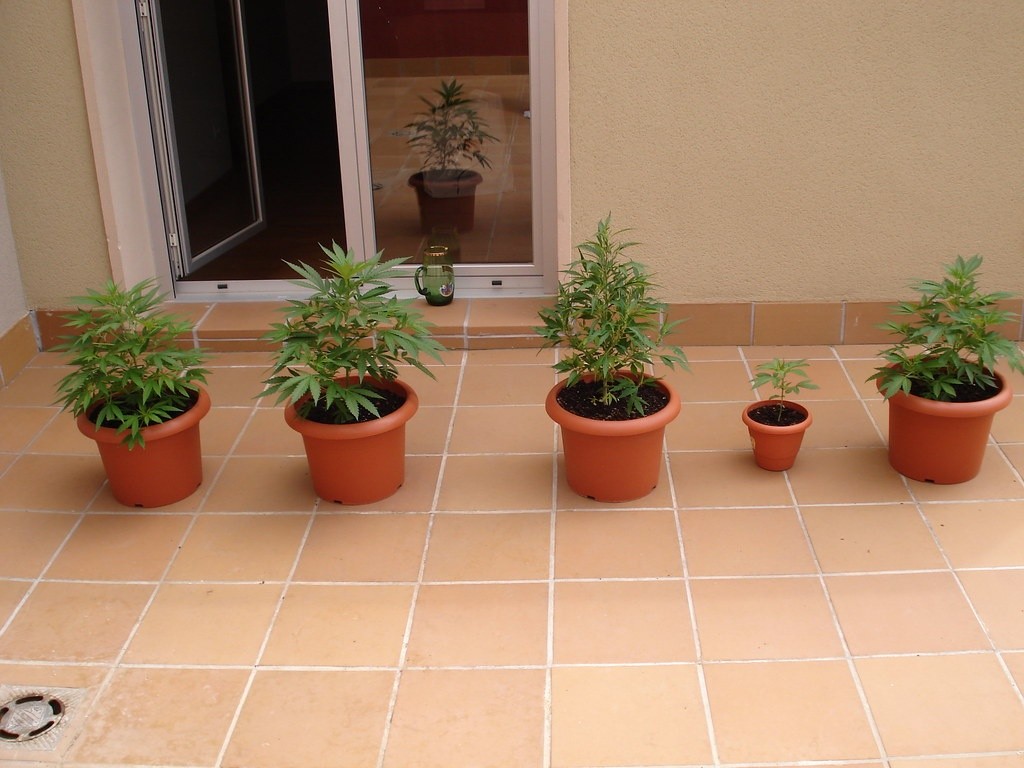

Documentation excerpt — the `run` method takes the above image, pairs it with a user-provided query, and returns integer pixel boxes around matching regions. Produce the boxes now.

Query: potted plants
[860,252,1024,485]
[742,357,821,471]
[531,210,694,501]
[402,78,502,234]
[44,275,219,508]
[252,239,452,504]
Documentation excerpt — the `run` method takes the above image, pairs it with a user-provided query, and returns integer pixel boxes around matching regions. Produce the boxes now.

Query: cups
[414,245,455,306]
